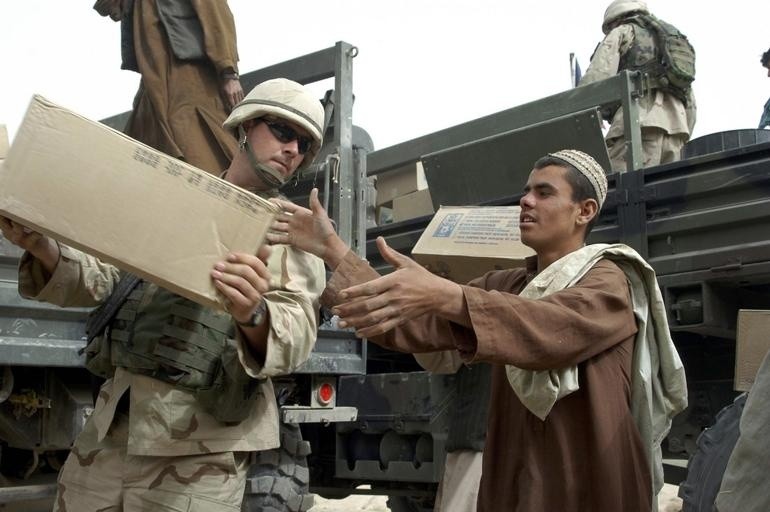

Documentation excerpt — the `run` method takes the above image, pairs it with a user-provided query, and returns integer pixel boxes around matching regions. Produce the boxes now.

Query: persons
[94,0,246,189]
[1,80,327,512]
[263,148,683,512]
[566,2,699,174]
[760,55,770,133]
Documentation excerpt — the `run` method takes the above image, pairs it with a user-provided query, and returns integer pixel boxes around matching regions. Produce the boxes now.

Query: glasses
[259,116,314,155]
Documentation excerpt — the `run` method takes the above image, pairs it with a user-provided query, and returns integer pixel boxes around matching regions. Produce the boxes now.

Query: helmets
[221,77,325,171]
[601,0,650,36]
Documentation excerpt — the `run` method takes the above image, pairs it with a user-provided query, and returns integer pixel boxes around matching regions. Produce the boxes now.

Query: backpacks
[634,13,697,91]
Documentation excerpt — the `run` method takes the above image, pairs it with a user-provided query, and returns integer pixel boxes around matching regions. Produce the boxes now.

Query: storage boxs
[374,161,429,205]
[373,205,392,225]
[0,93,282,310]
[730,306,770,393]
[391,188,439,226]
[411,203,540,286]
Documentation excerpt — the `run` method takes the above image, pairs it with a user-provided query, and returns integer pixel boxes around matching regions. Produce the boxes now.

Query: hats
[548,149,609,215]
